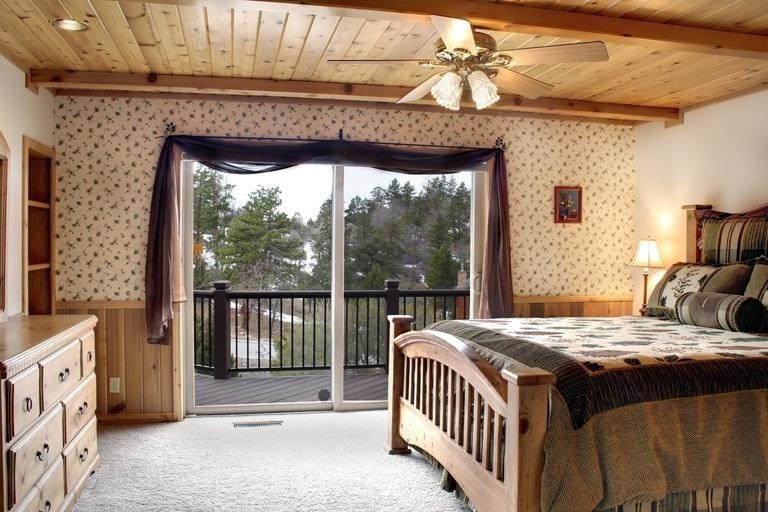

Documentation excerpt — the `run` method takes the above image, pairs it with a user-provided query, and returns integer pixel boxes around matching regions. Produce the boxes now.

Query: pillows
[643,260,753,327]
[697,216,768,265]
[675,290,764,333]
[744,255,768,332]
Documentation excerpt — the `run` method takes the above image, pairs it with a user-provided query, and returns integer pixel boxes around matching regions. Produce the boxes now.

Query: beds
[383,201,768,512]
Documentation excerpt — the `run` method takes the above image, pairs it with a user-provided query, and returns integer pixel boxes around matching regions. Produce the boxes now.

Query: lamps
[629,235,666,316]
[430,71,504,113]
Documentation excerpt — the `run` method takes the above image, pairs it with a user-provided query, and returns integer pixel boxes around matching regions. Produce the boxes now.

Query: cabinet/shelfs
[0,312,102,512]
[23,132,57,317]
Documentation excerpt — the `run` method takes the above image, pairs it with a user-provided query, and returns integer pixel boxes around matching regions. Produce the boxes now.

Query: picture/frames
[553,185,583,224]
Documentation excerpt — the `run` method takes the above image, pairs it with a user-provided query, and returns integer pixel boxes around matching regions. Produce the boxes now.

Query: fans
[325,12,610,116]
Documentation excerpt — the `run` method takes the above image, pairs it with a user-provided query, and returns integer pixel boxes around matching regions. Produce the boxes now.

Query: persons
[559,194,571,223]
